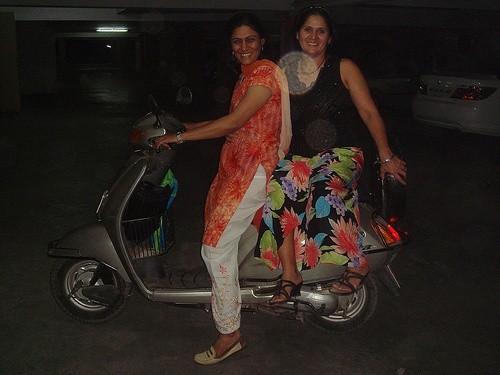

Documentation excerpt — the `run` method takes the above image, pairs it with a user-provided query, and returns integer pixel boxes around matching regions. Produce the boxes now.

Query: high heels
[329,270,370,296]
[269,278,303,305]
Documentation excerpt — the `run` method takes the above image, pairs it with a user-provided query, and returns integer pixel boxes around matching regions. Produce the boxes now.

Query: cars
[414,51,500,137]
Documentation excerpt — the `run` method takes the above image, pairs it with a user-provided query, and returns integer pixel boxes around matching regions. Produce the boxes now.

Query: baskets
[121,211,176,259]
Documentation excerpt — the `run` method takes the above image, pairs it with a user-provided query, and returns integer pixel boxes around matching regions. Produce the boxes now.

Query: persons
[268,5,409,305]
[152,18,293,365]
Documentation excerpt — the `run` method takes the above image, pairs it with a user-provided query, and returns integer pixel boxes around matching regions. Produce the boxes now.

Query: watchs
[175,131,183,144]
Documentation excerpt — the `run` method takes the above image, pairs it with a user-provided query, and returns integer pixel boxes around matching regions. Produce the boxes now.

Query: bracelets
[379,152,396,163]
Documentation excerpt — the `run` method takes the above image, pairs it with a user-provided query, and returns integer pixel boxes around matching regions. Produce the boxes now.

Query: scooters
[45,86,413,334]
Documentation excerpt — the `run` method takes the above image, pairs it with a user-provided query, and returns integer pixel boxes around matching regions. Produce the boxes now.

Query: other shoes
[194,333,247,365]
